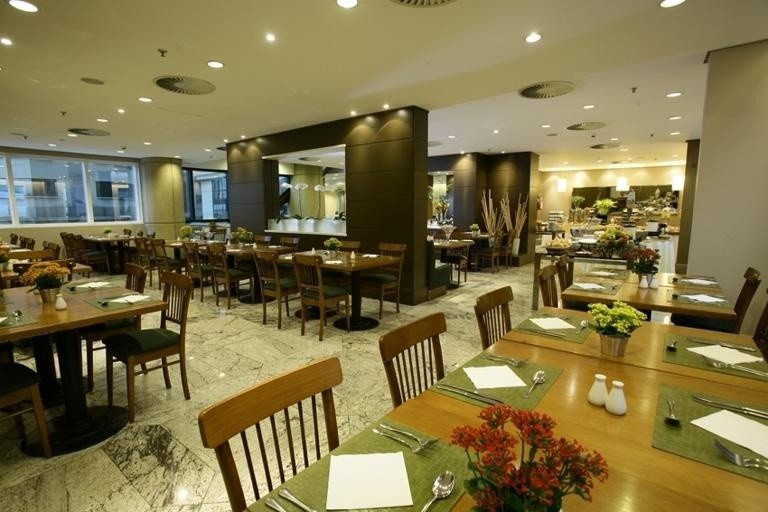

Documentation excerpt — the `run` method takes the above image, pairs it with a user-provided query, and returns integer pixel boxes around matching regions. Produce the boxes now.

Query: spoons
[575,319,588,335]
[522,369,547,399]
[666,339,678,350]
[96,300,109,306]
[419,470,456,511]
[664,396,680,421]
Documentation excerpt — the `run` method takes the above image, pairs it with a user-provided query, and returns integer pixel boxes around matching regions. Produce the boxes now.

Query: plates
[578,238,598,245]
[544,243,572,250]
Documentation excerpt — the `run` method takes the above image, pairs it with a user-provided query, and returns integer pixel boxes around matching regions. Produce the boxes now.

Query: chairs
[205,231,409,341]
[0,216,209,462]
[474,285,517,351]
[531,252,579,307]
[378,310,448,409]
[427,225,523,291]
[668,266,764,333]
[197,357,346,511]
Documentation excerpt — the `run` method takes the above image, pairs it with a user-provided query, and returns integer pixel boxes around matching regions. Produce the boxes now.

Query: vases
[595,331,631,357]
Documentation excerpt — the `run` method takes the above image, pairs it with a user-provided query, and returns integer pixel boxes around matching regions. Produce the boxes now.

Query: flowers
[272,181,345,224]
[580,299,648,338]
[448,402,613,512]
[427,184,453,223]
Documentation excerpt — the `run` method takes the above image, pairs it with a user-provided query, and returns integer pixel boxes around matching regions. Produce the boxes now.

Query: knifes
[518,326,567,339]
[685,336,758,354]
[436,379,506,406]
[692,394,767,421]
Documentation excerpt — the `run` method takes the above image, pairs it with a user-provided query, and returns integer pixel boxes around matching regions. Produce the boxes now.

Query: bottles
[177,236,181,242]
[649,275,659,289]
[192,236,197,243]
[639,276,648,288]
[350,251,356,259]
[606,380,627,416]
[311,247,316,254]
[226,240,231,246]
[54,293,68,310]
[252,242,258,249]
[586,373,608,407]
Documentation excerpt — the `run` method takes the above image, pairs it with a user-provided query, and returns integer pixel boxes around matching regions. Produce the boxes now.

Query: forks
[699,356,767,379]
[380,421,441,449]
[372,426,426,455]
[481,352,531,368]
[712,438,768,472]
